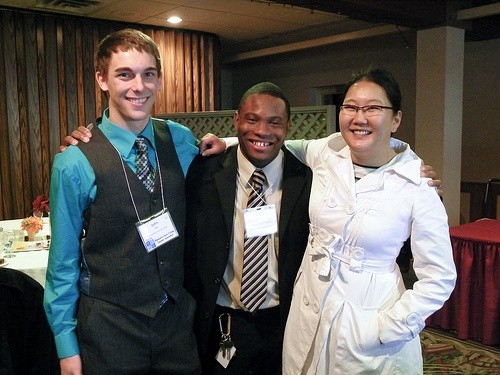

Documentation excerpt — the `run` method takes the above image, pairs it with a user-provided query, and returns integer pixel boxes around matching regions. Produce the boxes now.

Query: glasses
[339,103,395,117]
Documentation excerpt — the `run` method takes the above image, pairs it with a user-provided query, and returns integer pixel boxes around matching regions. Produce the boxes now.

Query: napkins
[32,195,50,217]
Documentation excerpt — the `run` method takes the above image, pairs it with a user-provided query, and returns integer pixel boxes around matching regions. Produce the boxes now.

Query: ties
[135,136,155,193]
[239,170,267,314]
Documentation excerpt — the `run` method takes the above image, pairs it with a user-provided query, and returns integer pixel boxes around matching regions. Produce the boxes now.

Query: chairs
[0,267,61,375]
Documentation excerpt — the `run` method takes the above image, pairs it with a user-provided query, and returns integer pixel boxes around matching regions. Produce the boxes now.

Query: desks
[426,218,500,347]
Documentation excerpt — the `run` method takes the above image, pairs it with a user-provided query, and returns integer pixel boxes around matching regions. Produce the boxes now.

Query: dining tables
[0,216,53,290]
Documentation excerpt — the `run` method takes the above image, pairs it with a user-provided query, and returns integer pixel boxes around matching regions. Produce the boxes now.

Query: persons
[57,81,444,375]
[200,64,457,375]
[43,29,201,374]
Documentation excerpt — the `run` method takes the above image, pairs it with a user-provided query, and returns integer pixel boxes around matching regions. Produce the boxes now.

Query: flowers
[19,215,44,235]
